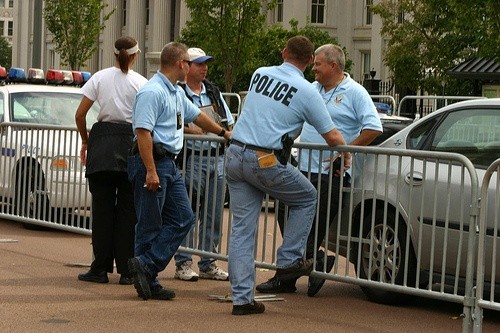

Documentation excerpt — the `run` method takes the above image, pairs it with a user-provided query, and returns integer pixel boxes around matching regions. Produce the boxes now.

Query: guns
[279,133,294,166]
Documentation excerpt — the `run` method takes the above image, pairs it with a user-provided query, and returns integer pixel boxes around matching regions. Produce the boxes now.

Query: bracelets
[218,129,226,137]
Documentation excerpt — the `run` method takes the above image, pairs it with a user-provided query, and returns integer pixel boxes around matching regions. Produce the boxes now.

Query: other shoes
[76,263,109,283]
[306,255,336,296]
[137,286,176,300]
[118,273,134,285]
[277,258,317,282]
[256,274,297,292]
[129,257,151,301]
[232,300,266,316]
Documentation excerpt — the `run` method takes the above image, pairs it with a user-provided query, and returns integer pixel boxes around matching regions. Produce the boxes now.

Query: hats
[186,47,212,64]
[113,37,142,56]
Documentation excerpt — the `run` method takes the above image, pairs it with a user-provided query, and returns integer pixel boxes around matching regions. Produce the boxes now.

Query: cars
[321,97,500,316]
[361,112,412,147]
[0,65,95,231]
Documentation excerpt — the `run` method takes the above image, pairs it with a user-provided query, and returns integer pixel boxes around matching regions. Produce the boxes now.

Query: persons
[75,37,150,283]
[225,35,356,313]
[254,43,384,294]
[127,42,236,300]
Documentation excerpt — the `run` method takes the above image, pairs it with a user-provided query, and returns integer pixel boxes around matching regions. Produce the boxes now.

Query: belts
[230,138,273,153]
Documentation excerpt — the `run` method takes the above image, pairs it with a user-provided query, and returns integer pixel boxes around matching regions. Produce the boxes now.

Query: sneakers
[172,259,199,281]
[197,260,230,280]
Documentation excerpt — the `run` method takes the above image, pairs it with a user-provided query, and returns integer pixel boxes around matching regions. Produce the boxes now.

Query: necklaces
[320,75,343,105]
